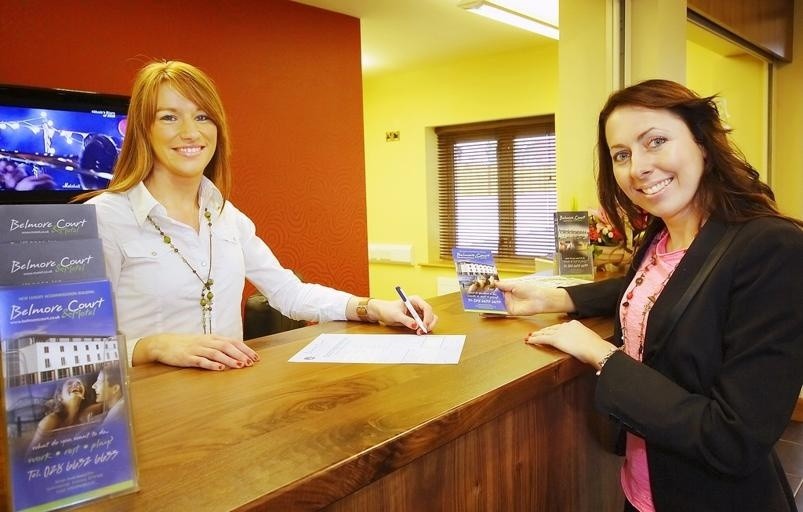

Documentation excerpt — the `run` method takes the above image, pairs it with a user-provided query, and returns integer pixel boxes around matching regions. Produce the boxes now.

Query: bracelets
[356,297,376,323]
[592,345,625,377]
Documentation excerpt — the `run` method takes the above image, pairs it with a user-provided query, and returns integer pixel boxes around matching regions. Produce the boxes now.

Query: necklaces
[619,229,688,362]
[148,206,214,335]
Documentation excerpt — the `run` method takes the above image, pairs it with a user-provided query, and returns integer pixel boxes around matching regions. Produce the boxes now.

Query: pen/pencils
[396,286,427,334]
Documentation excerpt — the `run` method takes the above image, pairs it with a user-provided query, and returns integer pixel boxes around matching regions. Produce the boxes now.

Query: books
[553,211,594,276]
[452,248,509,315]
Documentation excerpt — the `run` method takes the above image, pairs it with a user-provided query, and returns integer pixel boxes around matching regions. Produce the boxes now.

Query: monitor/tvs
[0,85,214,204]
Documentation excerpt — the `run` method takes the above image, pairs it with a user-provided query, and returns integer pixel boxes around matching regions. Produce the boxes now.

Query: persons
[26,361,126,451]
[467,273,500,292]
[479,79,803,512]
[72,60,438,371]
